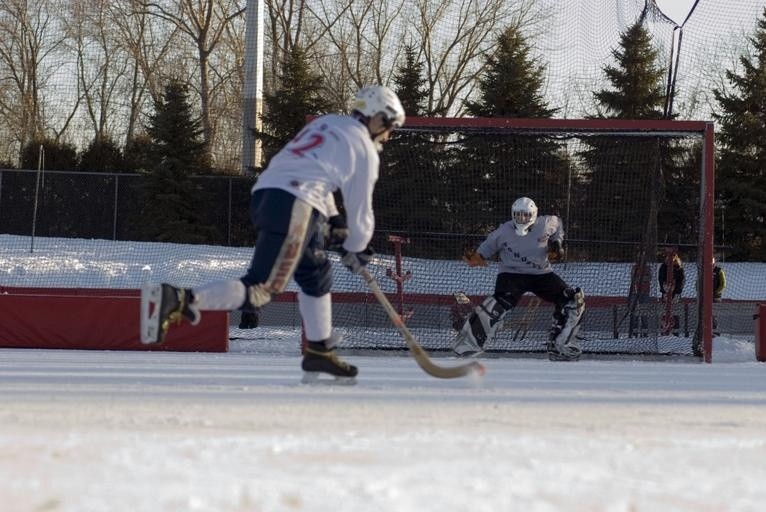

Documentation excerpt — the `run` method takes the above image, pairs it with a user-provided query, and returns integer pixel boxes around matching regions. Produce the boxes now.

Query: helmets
[352,85,406,129]
[510,196,538,229]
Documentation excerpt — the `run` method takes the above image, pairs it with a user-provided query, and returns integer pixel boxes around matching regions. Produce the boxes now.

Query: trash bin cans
[753,302,766,362]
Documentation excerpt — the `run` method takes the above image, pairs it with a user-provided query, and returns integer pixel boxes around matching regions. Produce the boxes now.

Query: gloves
[340,244,374,274]
[323,219,351,251]
[548,245,561,263]
[463,247,485,267]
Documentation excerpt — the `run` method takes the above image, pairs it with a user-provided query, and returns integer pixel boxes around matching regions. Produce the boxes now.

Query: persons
[447,196,586,360]
[657,247,685,338]
[627,248,653,339]
[153,81,407,378]
[695,255,728,338]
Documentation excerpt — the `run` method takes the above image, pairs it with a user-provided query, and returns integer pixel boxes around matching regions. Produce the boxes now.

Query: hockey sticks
[361,269,484,379]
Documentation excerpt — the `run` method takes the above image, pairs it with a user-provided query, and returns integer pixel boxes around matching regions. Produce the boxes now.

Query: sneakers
[631,329,649,339]
[546,341,582,359]
[659,328,681,337]
[452,336,479,357]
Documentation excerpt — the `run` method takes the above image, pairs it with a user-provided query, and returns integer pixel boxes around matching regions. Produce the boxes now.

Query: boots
[301,334,358,377]
[155,282,200,344]
